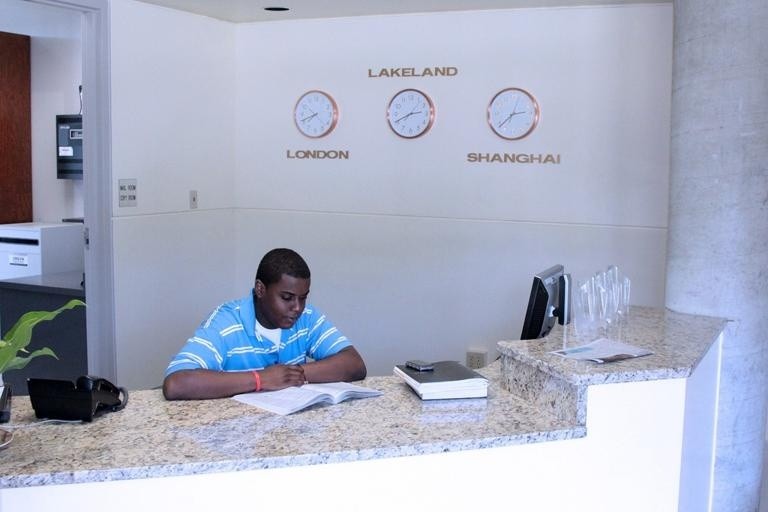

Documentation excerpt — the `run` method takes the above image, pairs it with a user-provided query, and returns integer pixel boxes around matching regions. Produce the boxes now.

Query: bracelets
[250,367,262,393]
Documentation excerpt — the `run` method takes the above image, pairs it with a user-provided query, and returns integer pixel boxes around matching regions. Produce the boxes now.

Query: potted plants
[0,296,87,424]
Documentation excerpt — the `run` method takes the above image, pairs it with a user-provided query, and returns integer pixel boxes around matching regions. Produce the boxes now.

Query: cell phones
[406,360,433,371]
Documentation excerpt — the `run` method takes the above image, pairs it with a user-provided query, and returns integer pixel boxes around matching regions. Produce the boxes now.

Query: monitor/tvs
[520,264,572,340]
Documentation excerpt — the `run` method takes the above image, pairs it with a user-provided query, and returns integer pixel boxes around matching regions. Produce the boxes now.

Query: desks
[0,268,87,397]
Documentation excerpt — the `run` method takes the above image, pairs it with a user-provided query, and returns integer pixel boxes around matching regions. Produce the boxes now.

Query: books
[390,358,491,402]
[226,380,385,420]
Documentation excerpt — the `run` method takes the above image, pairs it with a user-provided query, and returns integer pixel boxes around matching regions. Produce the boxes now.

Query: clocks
[484,85,542,144]
[290,90,341,140]
[383,86,437,142]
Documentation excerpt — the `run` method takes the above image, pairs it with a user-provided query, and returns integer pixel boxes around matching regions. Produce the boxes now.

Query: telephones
[26,376,121,422]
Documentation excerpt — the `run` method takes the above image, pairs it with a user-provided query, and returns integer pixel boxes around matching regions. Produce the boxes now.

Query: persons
[159,246,369,403]
